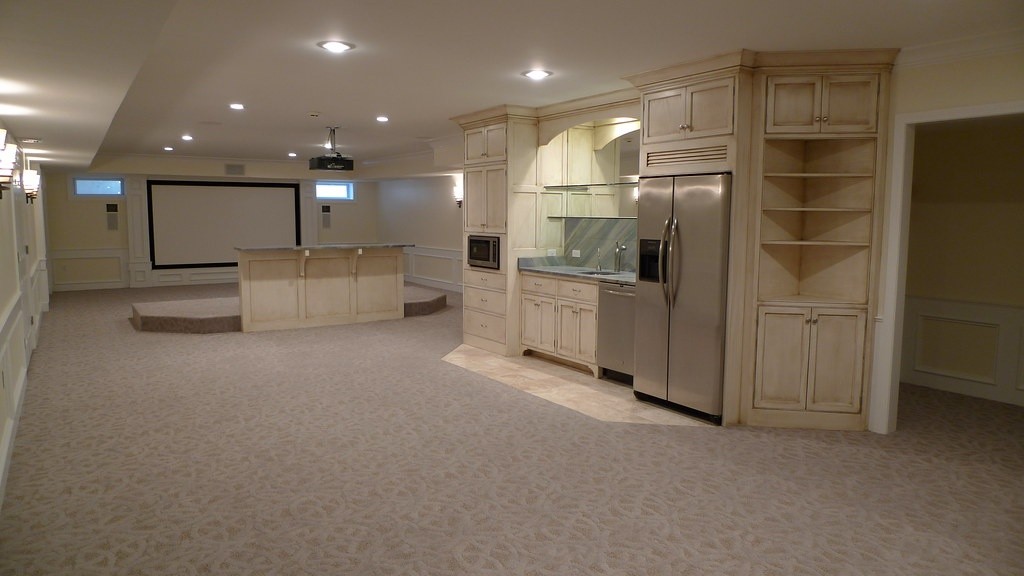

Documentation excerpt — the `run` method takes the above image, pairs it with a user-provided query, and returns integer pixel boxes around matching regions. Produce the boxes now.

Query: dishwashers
[597,282,635,387]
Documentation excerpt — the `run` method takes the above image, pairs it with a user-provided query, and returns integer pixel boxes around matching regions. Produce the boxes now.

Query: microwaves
[467,234,500,269]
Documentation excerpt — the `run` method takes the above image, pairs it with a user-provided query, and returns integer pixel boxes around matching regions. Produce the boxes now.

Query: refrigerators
[632,174,732,426]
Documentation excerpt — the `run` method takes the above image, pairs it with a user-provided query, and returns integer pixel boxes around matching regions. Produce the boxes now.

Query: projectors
[309,156,354,171]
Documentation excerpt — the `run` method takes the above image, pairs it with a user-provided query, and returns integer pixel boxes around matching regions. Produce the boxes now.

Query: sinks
[577,272,621,275]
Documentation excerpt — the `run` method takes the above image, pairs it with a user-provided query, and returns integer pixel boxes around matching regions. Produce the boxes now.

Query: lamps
[0,128,40,204]
[453,186,463,208]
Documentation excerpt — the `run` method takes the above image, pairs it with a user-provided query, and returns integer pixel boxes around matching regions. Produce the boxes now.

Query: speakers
[322,205,330,213]
[107,203,118,212]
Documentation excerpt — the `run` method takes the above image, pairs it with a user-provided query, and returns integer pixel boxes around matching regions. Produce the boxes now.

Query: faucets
[614,240,627,272]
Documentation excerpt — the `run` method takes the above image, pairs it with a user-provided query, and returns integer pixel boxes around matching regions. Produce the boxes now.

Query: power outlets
[546,248,557,257]
[572,250,581,257]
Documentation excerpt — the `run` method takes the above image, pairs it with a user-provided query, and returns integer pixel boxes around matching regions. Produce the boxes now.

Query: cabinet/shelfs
[765,73,878,134]
[462,164,536,235]
[640,73,751,144]
[462,121,538,166]
[760,172,874,246]
[751,305,869,414]
[555,276,603,379]
[518,271,556,379]
[462,267,520,357]
